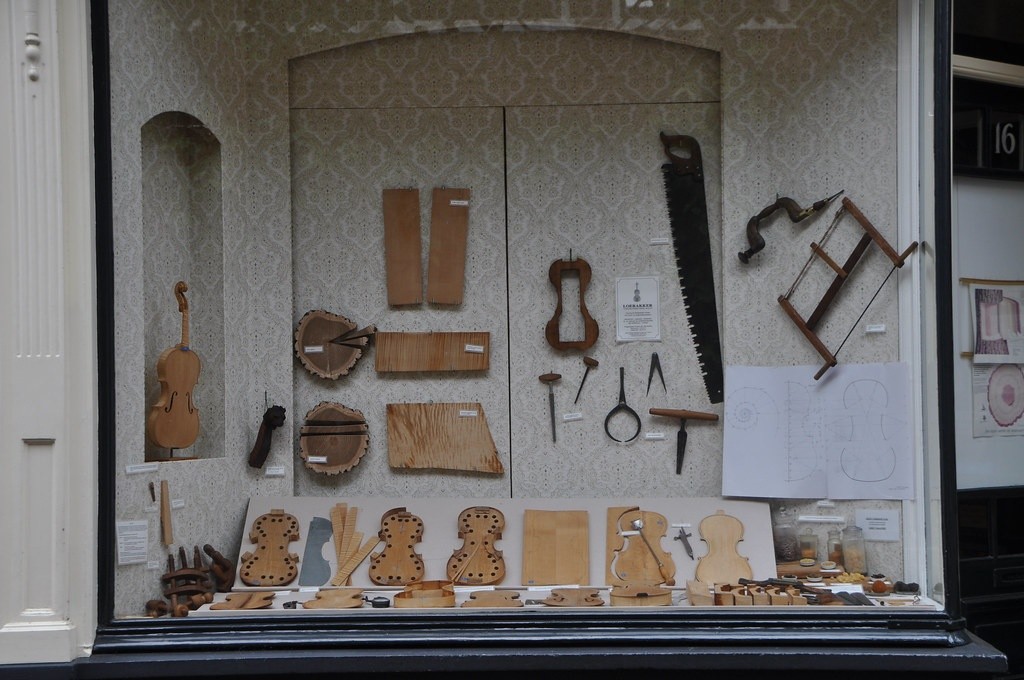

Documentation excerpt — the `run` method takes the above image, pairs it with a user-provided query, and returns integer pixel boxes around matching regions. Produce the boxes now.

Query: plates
[800,560,815,566]
[821,560,837,570]
[869,574,885,583]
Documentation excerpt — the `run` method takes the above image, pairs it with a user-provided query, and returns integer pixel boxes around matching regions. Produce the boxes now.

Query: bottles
[773,504,800,562]
[828,528,841,565]
[800,533,819,559]
[841,525,867,576]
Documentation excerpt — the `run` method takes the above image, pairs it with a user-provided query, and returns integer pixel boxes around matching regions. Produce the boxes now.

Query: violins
[146,280,200,450]
